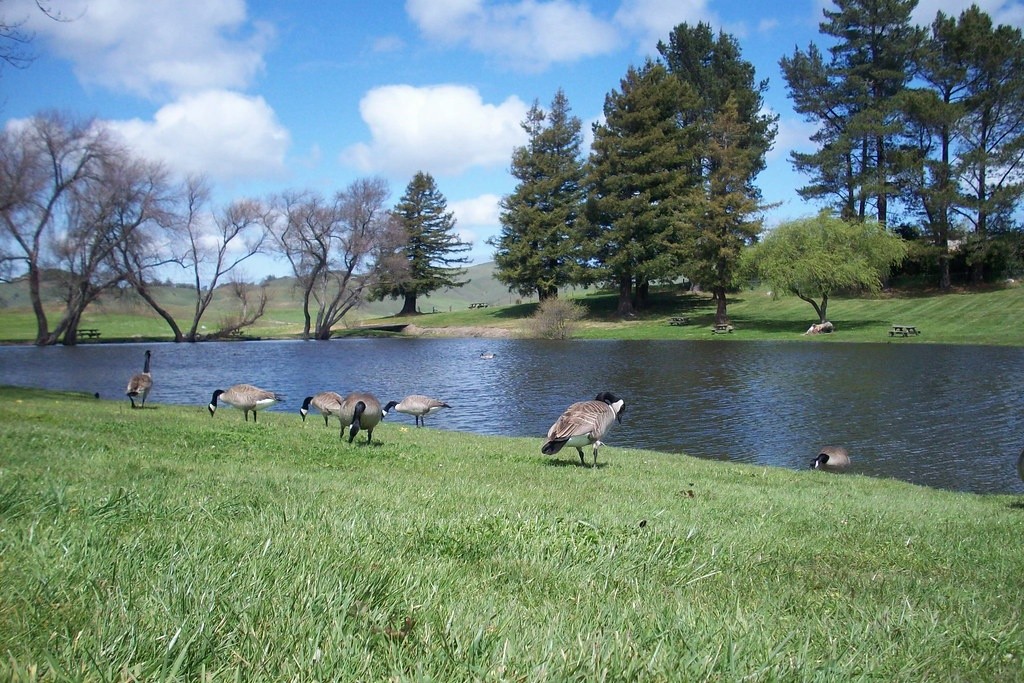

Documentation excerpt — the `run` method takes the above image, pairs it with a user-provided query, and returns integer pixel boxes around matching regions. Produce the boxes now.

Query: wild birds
[809,446,850,471]
[299,392,345,428]
[480,352,496,358]
[338,393,382,444]
[124,350,155,410]
[380,394,452,429]
[208,383,285,423]
[541,390,627,469]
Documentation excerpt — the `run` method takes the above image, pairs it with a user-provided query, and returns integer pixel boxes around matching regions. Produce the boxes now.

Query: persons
[804,319,835,336]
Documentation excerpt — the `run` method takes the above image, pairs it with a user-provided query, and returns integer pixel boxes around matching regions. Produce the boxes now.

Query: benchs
[904,330,921,334]
[669,320,684,324]
[888,331,908,336]
[712,326,734,332]
[680,319,691,321]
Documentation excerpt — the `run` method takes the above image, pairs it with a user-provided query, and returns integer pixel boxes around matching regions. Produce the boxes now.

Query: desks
[717,323,727,329]
[671,316,689,324]
[892,326,918,335]
